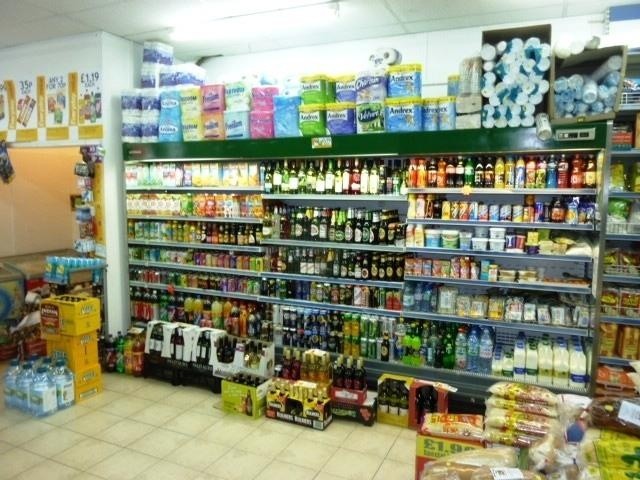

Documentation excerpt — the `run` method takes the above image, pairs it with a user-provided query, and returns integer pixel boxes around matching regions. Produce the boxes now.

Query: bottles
[548,195,565,223]
[408,153,598,188]
[45,256,100,283]
[264,158,410,283]
[407,193,442,219]
[79,86,101,125]
[149,323,367,424]
[166,219,261,245]
[402,282,442,312]
[128,220,134,239]
[376,318,586,424]
[98,329,146,377]
[130,287,266,339]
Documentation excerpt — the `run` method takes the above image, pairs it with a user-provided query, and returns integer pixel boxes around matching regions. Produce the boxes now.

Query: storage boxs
[46,331,98,371]
[72,364,103,402]
[39,294,101,335]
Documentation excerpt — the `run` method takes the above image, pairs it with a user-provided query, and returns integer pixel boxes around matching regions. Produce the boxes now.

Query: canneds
[272,303,397,362]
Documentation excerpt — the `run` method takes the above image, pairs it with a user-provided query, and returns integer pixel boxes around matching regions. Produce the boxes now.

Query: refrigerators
[0,248,80,358]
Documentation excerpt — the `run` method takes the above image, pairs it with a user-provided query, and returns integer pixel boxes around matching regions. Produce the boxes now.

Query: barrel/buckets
[26,353,42,374]
[553,344,569,386]
[39,357,54,372]
[15,362,37,411]
[50,360,75,410]
[568,335,581,343]
[30,367,58,418]
[539,334,552,340]
[554,336,567,341]
[538,341,553,383]
[3,358,23,409]
[553,339,567,346]
[568,340,583,350]
[569,345,586,389]
[538,337,552,348]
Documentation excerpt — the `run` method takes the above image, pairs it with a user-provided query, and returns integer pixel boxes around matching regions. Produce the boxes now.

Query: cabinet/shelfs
[124,157,271,380]
[400,151,602,395]
[596,151,639,401]
[261,156,404,388]
